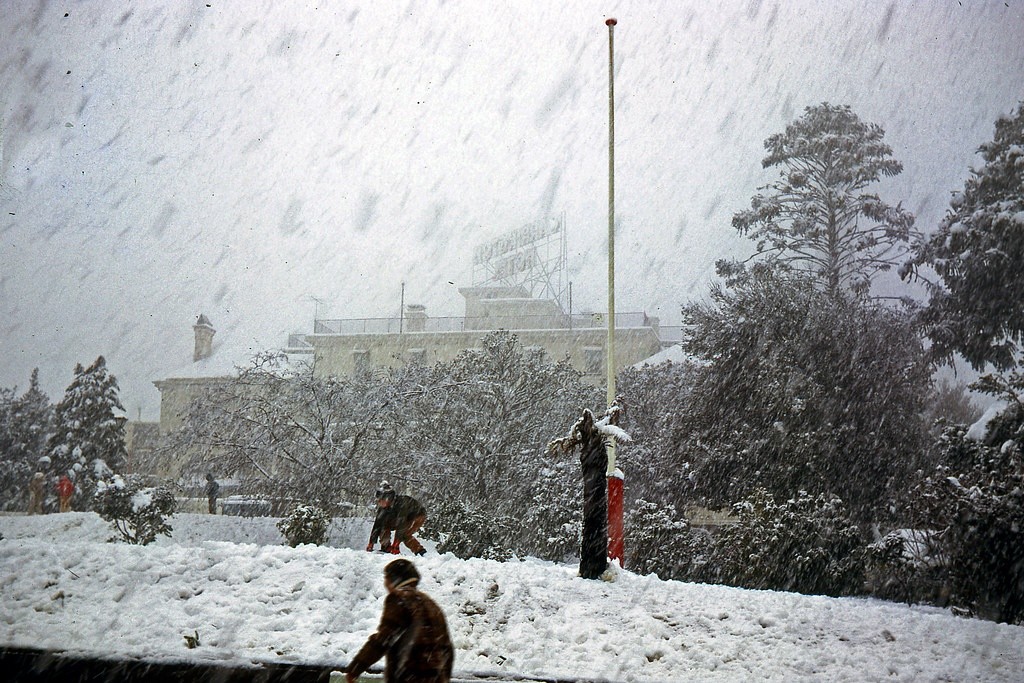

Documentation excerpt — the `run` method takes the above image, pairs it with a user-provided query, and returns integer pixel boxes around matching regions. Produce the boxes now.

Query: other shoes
[415,549,427,556]
[378,543,391,553]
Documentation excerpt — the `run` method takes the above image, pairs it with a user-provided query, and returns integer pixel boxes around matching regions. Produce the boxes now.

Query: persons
[56,475,74,513]
[27,472,48,516]
[205,473,220,515]
[345,559,455,683]
[366,480,428,557]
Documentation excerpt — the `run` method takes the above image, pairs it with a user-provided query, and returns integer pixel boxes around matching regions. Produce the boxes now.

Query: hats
[376,481,395,498]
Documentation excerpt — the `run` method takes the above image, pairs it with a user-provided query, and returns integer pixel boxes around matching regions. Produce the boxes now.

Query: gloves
[366,542,374,551]
[390,539,400,555]
